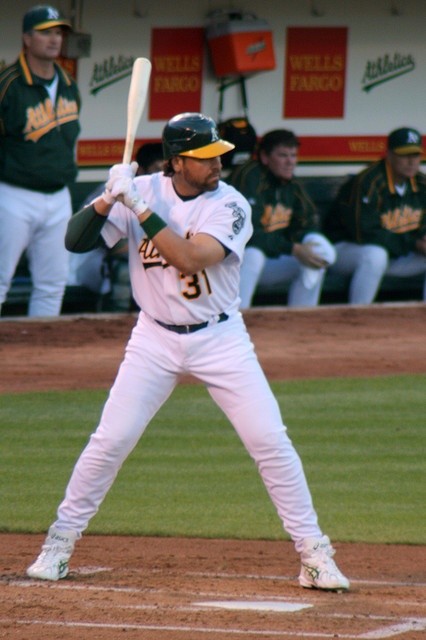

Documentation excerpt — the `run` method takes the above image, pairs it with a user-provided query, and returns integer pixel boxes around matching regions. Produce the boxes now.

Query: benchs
[1,176,425,303]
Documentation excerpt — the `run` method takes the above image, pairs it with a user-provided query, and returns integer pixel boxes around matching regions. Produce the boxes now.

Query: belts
[155,312,228,334]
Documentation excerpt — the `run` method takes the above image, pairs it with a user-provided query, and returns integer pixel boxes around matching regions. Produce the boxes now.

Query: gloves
[102,161,139,205]
[105,176,149,216]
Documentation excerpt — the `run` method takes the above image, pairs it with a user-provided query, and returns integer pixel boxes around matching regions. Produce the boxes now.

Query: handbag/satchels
[218,117,256,168]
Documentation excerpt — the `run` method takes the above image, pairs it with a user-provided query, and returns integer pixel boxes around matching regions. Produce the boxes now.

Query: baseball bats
[116,58,152,201]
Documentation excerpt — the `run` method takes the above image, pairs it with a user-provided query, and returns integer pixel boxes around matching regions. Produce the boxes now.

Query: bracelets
[139,212,167,239]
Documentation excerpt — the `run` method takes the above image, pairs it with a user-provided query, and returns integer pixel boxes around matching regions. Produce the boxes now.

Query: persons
[0,5,82,316]
[66,144,166,293]
[27,112,351,589]
[324,127,426,304]
[224,128,337,309]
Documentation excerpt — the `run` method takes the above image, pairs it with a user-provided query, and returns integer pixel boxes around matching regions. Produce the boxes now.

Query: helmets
[162,113,235,160]
[23,6,73,31]
[388,128,424,155]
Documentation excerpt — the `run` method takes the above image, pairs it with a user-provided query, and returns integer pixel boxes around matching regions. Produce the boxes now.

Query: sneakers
[299,537,350,590]
[26,525,77,581]
